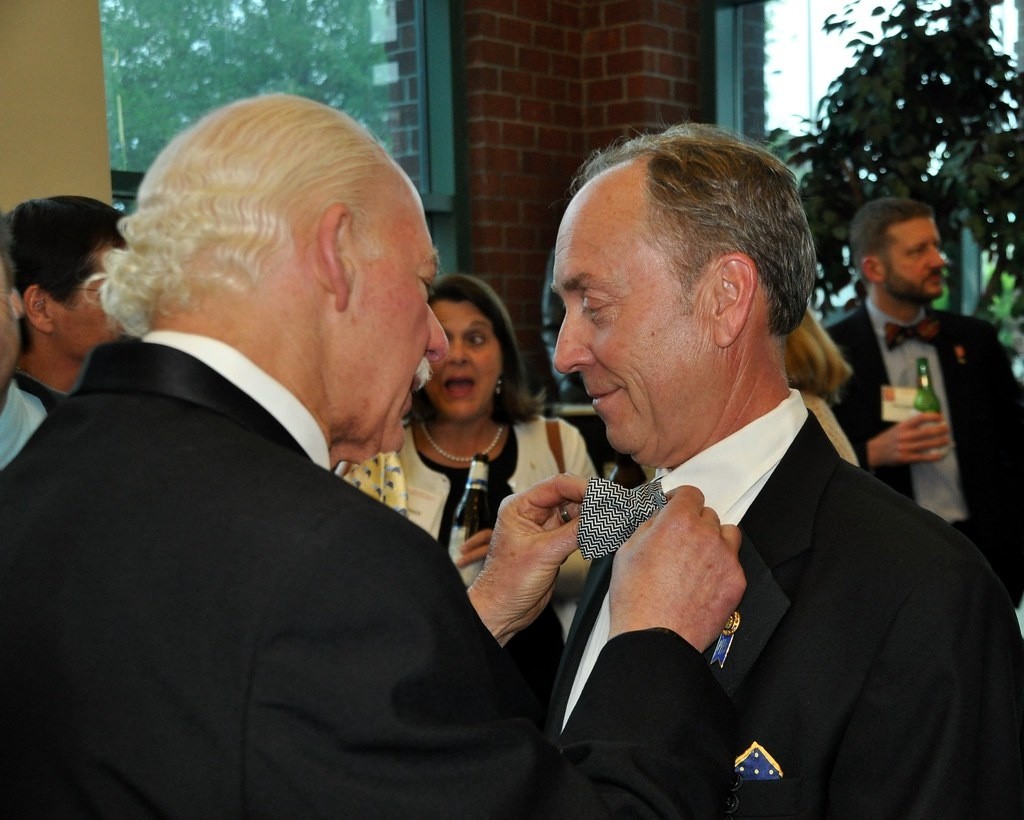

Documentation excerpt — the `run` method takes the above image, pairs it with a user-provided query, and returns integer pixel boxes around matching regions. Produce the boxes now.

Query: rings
[559,504,571,524]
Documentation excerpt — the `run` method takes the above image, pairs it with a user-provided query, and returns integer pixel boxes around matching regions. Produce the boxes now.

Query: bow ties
[884,316,943,351]
[577,478,668,561]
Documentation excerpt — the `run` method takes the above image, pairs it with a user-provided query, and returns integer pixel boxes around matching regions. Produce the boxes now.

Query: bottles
[913,356,949,456]
[444,452,497,590]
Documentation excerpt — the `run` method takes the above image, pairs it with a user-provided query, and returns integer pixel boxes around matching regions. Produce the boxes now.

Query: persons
[826,197,1023,608]
[786,311,858,470]
[0,94,748,819]
[398,274,599,710]
[0,196,125,468]
[553,123,1024,819]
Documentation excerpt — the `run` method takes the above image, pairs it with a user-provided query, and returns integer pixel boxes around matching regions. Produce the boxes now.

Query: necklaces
[420,422,502,461]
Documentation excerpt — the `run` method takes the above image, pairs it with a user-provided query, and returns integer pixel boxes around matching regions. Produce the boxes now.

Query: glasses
[44,271,108,307]
